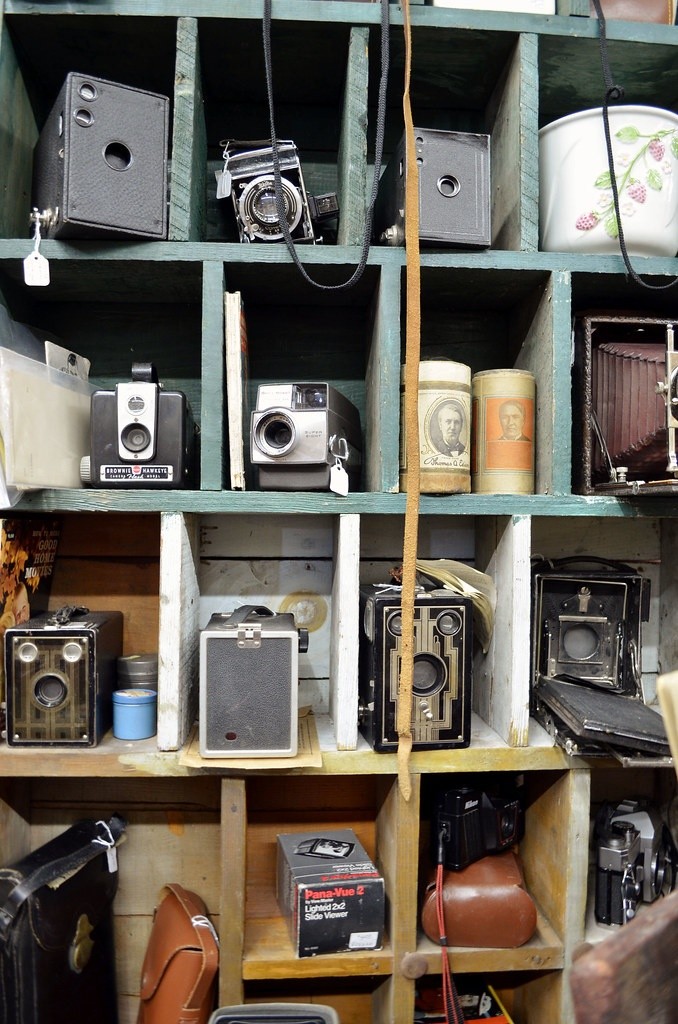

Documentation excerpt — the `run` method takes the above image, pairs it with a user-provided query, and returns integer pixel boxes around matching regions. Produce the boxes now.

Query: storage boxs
[270,829,385,960]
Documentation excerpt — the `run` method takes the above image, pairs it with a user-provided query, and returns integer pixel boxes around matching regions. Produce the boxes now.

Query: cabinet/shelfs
[0,0,678,1024]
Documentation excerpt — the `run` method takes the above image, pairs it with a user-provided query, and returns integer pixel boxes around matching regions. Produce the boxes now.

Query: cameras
[595,795,678,927]
[2,70,524,958]
[533,562,652,702]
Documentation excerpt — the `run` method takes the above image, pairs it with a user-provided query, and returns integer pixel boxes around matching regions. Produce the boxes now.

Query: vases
[535,105,678,258]
